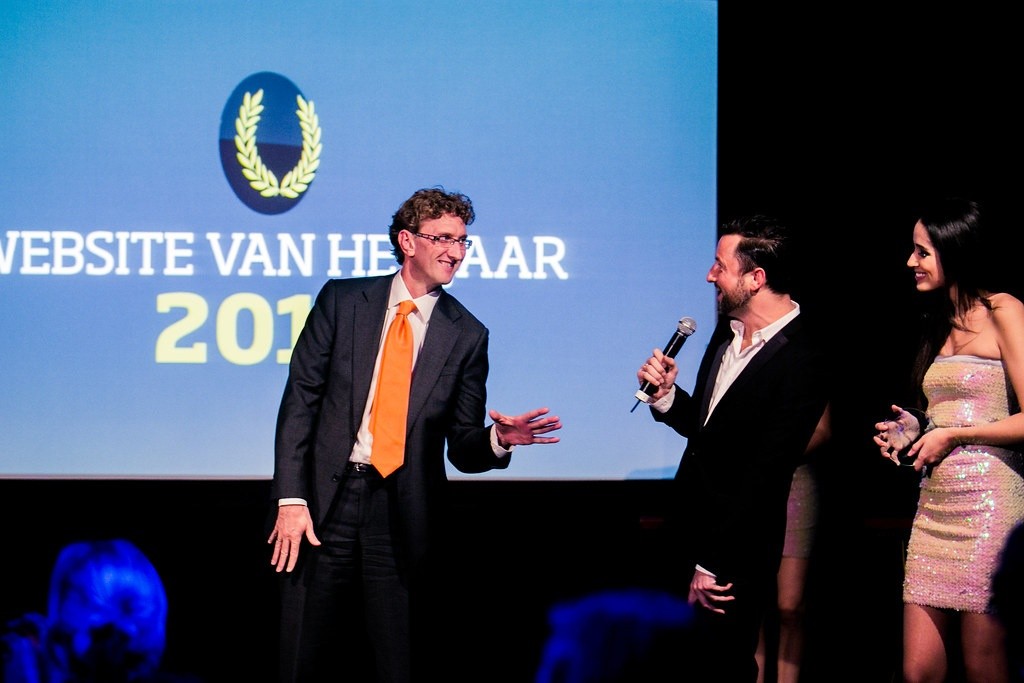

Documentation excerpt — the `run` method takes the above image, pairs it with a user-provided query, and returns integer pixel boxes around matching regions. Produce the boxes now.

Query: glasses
[414,231,472,250]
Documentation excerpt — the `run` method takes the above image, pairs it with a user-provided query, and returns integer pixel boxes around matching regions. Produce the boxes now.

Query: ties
[368,300,419,478]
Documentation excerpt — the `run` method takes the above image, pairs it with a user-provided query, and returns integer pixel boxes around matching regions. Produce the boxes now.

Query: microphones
[630,317,697,412]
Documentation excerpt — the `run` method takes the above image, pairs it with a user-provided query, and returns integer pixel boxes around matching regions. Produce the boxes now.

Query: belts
[344,460,380,477]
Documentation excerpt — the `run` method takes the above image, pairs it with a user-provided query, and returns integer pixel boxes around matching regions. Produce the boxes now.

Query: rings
[644,364,649,371]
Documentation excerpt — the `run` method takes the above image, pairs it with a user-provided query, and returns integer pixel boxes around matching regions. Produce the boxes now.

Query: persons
[9,538,168,683]
[540,596,740,683]
[753,403,831,683]
[268,186,562,683]
[873,202,1024,683]
[639,214,839,683]
